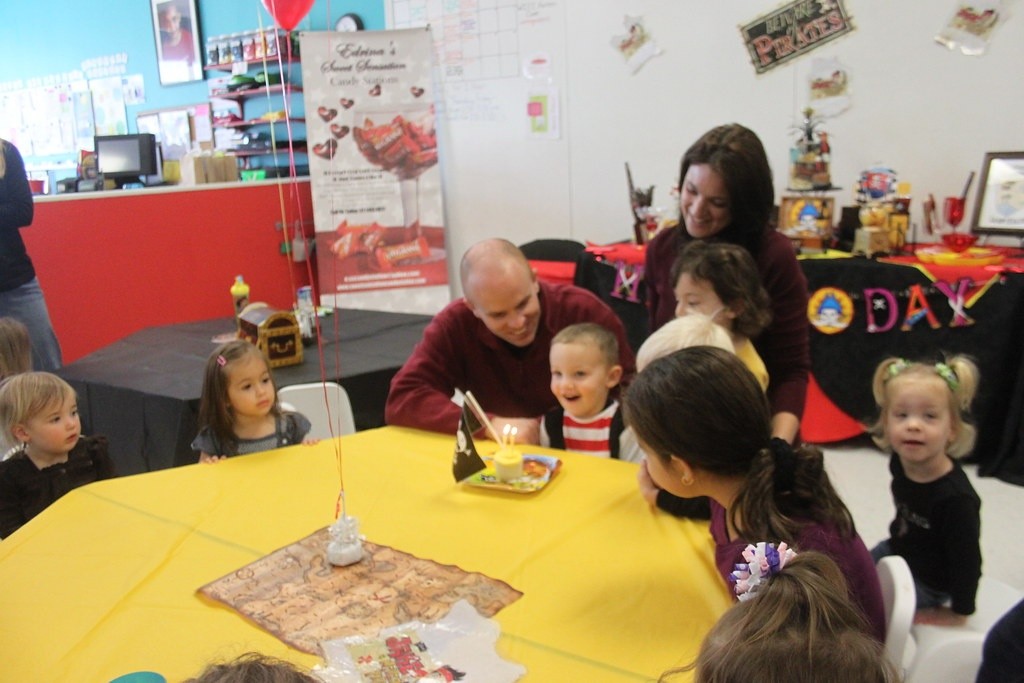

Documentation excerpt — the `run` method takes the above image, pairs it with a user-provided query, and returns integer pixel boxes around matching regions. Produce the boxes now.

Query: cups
[163,161,180,184]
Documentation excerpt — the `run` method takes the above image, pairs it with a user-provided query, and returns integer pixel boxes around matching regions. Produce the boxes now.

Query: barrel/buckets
[241,170,266,181]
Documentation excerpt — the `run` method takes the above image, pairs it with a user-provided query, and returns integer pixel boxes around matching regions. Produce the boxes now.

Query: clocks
[335,14,363,32]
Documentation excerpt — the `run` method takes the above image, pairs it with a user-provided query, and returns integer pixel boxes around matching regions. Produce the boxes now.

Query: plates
[469,462,550,493]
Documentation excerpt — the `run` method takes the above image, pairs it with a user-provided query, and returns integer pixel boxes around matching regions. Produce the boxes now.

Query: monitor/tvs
[94,134,157,189]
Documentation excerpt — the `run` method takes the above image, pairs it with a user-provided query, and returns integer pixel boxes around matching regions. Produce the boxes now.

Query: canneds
[206,25,287,65]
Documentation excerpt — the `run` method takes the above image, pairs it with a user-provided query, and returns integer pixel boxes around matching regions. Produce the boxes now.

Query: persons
[385,238,637,447]
[642,124,811,449]
[670,240,769,393]
[0,138,64,373]
[191,340,320,463]
[691,541,902,683]
[622,314,886,654]
[157,2,195,64]
[867,358,982,626]
[533,323,646,463]
[0,317,119,540]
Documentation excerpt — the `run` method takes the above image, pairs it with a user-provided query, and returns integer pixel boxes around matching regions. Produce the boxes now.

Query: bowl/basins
[942,233,978,253]
[28,181,44,195]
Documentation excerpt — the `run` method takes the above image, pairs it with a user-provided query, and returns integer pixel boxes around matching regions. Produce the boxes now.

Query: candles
[502,424,511,456]
[509,427,518,457]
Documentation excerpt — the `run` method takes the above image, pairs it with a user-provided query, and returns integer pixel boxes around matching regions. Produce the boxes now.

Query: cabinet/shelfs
[203,54,309,181]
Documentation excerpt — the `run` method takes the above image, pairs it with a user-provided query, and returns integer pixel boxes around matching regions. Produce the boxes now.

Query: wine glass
[944,198,964,234]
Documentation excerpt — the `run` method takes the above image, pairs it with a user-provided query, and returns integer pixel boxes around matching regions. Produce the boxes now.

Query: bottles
[231,276,250,329]
[206,25,300,66]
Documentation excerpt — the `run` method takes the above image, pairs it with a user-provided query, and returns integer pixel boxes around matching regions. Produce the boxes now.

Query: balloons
[261,0,315,31]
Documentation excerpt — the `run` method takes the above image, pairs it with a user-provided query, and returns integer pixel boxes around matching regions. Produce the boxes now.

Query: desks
[0,426,735,683]
[53,307,436,481]
[588,237,1024,488]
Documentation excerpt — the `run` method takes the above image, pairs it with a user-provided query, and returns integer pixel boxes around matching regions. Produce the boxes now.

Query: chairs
[518,237,588,291]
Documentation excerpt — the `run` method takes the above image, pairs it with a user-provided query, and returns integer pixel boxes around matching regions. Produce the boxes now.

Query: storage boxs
[236,300,305,370]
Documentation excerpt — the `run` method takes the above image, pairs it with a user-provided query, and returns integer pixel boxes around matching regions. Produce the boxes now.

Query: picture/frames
[149,0,205,88]
[970,151,1024,247]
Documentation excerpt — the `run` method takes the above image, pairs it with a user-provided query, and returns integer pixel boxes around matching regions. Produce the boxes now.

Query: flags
[452,398,487,483]
